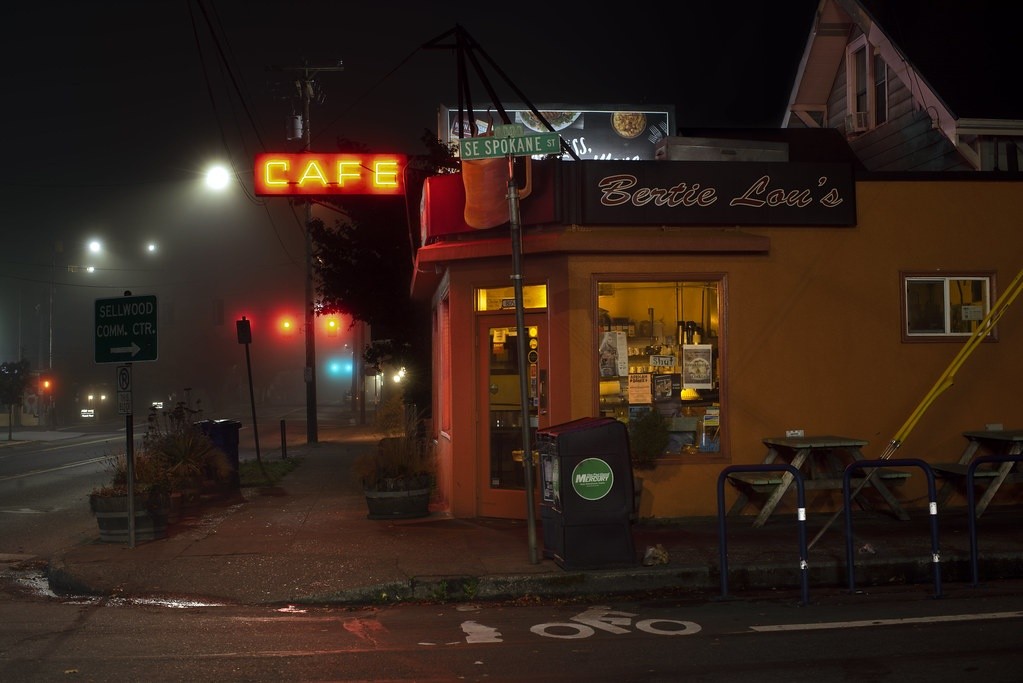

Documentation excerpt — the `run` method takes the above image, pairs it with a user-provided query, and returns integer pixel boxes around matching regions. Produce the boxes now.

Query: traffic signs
[92,294,160,366]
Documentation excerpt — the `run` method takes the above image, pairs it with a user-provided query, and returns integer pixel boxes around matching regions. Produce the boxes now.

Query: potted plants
[92,385,212,542]
[362,394,436,518]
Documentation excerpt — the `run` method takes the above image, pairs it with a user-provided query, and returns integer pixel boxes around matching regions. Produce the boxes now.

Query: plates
[518,111,581,133]
[610,113,647,138]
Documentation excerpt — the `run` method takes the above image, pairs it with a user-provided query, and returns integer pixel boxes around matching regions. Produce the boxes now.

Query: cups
[629,363,654,375]
[677,320,685,346]
[627,347,647,356]
[687,321,695,344]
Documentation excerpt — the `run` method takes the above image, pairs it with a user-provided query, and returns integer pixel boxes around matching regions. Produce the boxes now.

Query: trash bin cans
[191,418,243,489]
[535,415,638,570]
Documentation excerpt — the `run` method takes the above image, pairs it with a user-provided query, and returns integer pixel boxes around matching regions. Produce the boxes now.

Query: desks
[725,433,913,528]
[489,409,538,489]
[929,429,1023,534]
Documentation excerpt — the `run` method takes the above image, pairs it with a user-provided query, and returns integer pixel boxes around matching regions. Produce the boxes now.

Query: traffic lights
[327,358,356,375]
[268,305,350,340]
[38,370,57,396]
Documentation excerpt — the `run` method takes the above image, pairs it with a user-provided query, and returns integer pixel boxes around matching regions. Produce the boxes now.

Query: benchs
[928,460,1023,487]
[726,466,912,491]
